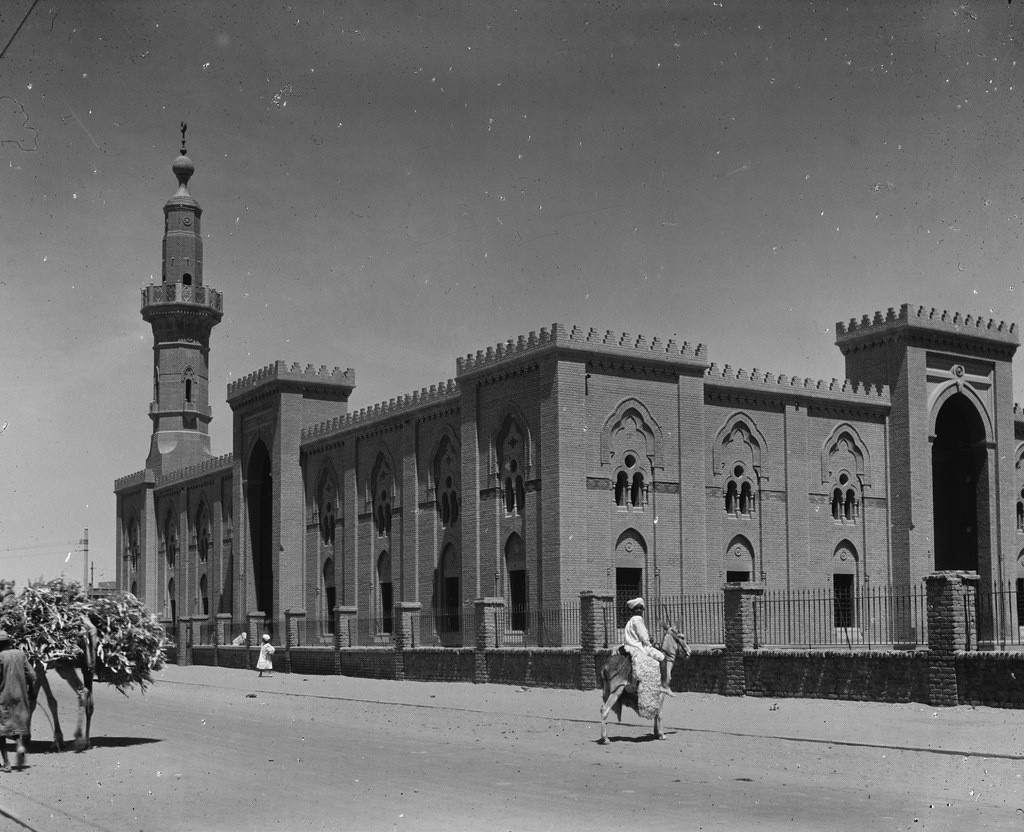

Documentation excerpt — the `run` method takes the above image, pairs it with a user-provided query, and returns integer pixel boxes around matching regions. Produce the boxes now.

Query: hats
[263,634,270,641]
[626,598,645,609]
[0,631,9,640]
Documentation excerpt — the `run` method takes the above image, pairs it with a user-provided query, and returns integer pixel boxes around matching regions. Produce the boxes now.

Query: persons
[624,597,674,697]
[256,634,275,677]
[0,630,37,772]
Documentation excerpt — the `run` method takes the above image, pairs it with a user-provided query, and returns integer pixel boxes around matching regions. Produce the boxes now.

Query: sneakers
[660,686,676,697]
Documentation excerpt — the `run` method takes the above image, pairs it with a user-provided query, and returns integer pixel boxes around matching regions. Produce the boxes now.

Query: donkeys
[600,615,692,745]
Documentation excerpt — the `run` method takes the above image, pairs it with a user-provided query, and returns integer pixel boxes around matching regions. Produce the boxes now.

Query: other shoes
[0,765,12,773]
[267,674,272,677]
[258,673,262,677]
[17,746,25,766]
